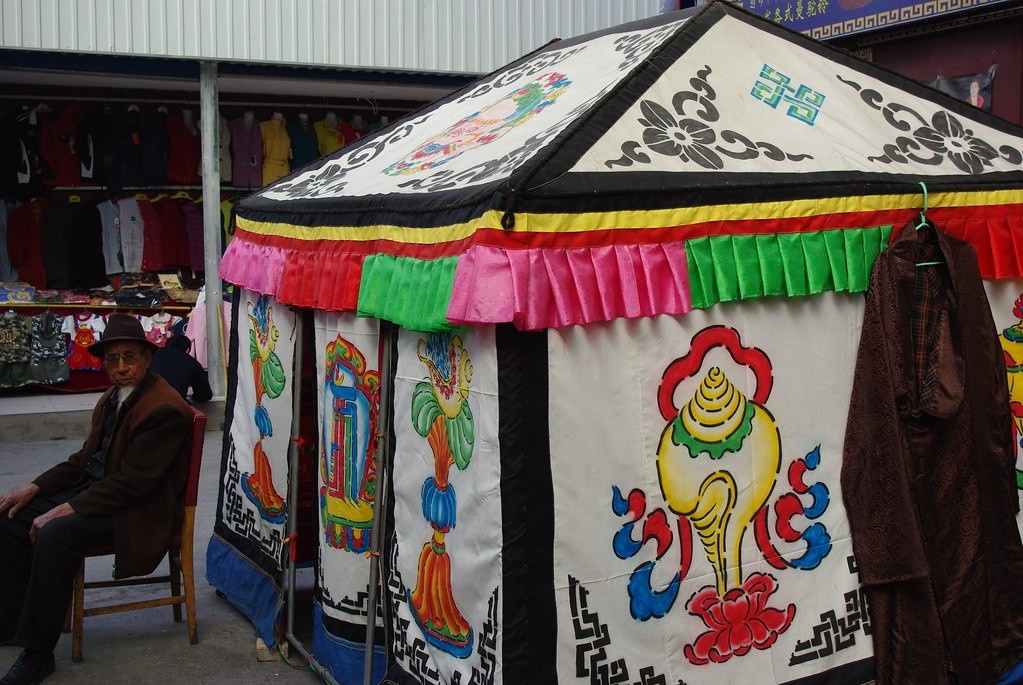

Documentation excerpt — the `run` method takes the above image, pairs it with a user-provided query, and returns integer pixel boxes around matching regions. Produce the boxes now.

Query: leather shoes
[0,648,55,685]
[0,622,25,647]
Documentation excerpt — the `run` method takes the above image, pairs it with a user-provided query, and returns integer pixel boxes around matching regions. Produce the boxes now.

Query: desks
[0,302,196,393]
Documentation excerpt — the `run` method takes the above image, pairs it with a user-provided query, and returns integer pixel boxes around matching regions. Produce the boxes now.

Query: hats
[87,314,161,358]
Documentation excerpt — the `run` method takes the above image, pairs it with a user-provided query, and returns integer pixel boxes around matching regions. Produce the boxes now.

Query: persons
[0,315,195,685]
[150,335,213,403]
[966,80,984,108]
[164,110,390,188]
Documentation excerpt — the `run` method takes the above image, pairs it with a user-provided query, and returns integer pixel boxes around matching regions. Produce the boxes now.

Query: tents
[205,0,1023,685]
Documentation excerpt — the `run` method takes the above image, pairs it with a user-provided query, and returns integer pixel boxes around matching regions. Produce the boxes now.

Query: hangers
[22,94,170,113]
[107,306,124,316]
[31,184,242,204]
[914,181,947,267]
[79,304,89,316]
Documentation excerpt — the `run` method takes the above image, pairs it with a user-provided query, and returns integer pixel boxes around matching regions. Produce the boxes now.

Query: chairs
[62,405,207,664]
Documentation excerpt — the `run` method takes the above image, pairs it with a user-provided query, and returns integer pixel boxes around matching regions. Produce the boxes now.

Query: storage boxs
[0,281,36,302]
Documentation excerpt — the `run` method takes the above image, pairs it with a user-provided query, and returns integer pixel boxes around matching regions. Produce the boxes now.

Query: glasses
[101,347,147,361]
